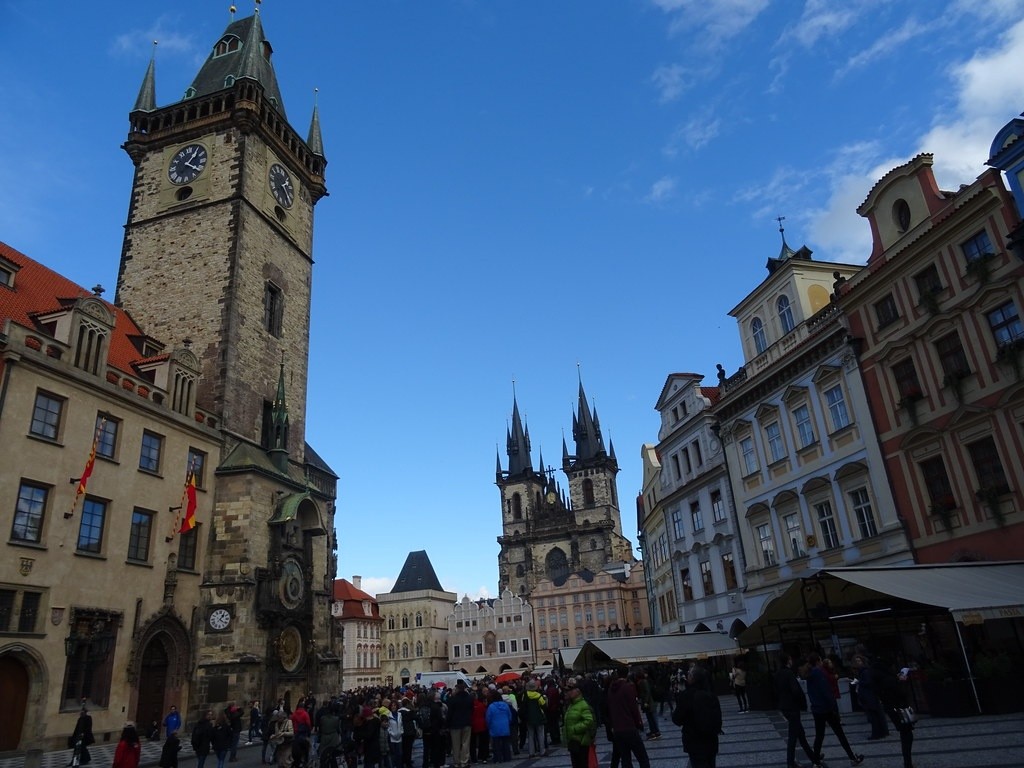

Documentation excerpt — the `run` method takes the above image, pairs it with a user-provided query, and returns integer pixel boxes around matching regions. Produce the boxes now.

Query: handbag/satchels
[894,705,919,725]
[588,744,598,768]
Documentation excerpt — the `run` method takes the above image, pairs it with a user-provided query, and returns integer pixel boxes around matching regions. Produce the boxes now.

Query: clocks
[166,143,208,187]
[204,601,237,635]
[269,164,296,208]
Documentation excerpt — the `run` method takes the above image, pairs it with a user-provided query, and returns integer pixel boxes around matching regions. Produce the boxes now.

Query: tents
[552,652,560,669]
[571,630,751,673]
[558,647,582,669]
[733,557,1024,712]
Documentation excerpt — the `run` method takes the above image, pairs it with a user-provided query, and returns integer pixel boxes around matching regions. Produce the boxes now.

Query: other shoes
[229,757,238,762]
[812,761,828,768]
[738,710,744,712]
[548,740,562,745]
[866,736,883,740]
[744,709,748,711]
[435,751,551,768]
[850,752,865,766]
[819,753,825,760]
[647,731,661,737]
[646,733,656,741]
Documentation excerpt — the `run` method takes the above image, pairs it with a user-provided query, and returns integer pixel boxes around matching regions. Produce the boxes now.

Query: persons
[65,660,921,768]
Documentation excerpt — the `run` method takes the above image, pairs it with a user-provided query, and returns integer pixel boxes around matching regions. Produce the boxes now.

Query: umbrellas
[435,681,446,687]
[494,671,521,683]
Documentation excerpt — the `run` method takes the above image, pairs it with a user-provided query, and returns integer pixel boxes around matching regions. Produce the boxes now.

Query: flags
[177,473,196,534]
[78,449,96,495]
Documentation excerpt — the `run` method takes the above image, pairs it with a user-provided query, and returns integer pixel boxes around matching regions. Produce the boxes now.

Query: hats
[526,680,538,690]
[562,677,580,690]
[503,686,513,693]
[123,721,136,728]
[362,707,374,717]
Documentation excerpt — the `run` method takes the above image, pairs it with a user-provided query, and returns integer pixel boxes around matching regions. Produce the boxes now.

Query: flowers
[941,494,956,506]
[901,384,921,398]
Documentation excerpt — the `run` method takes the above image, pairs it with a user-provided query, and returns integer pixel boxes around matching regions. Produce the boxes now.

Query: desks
[797,677,853,714]
